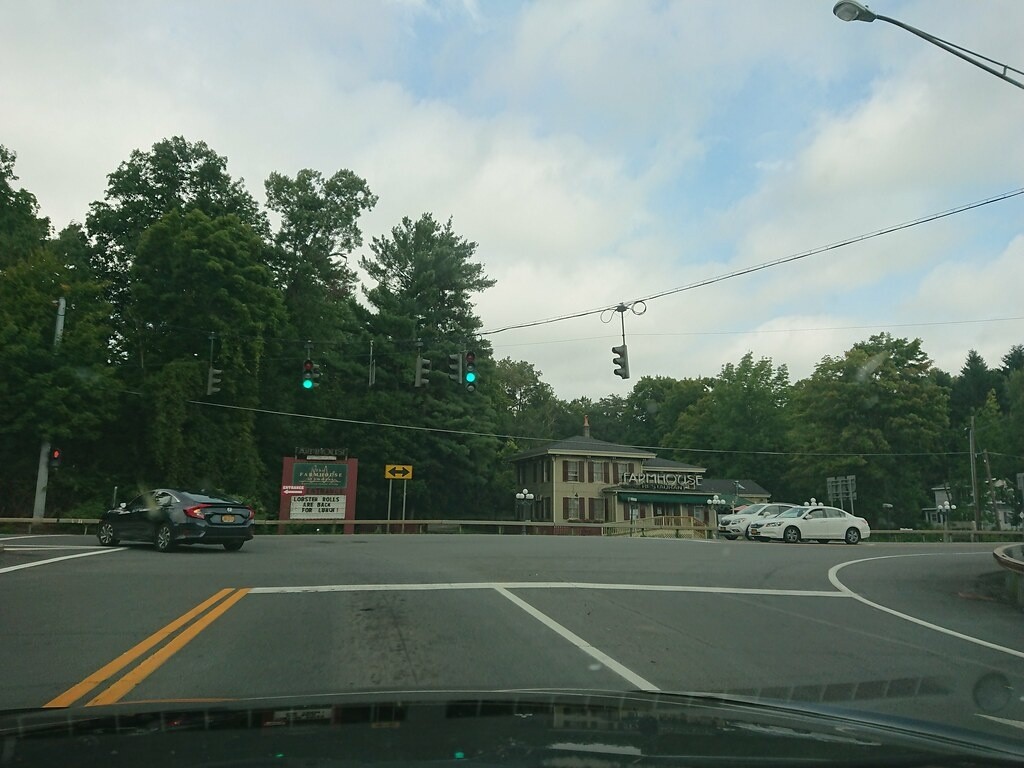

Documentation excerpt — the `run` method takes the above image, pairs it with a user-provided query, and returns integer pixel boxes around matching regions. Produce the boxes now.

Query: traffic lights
[302,360,320,390]
[415,357,430,388]
[448,354,462,385]
[612,345,630,379]
[465,351,476,393]
[49,449,64,467]
[207,368,222,396]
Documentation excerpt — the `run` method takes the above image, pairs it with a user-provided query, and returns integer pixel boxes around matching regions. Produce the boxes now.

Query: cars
[748,506,870,544]
[97,489,255,552]
[718,504,801,541]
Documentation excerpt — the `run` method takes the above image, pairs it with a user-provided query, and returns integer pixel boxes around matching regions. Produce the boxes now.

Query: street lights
[706,495,725,539]
[833,0,1024,89]
[516,489,534,535]
[938,501,956,542]
